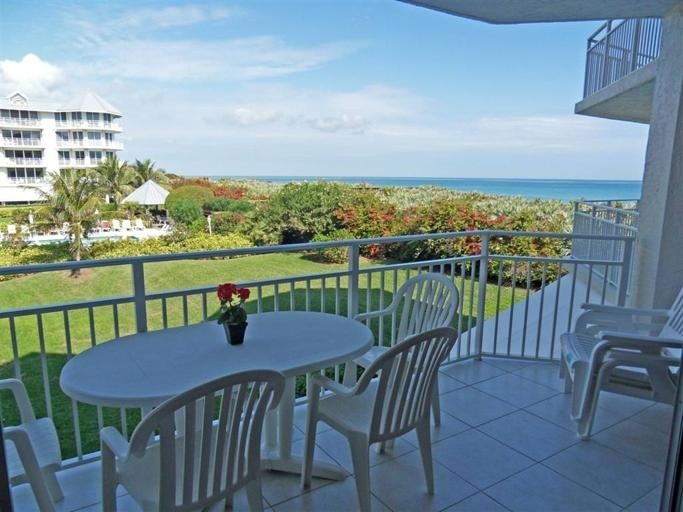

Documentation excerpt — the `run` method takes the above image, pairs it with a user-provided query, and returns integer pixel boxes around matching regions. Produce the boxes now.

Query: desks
[58,309,375,481]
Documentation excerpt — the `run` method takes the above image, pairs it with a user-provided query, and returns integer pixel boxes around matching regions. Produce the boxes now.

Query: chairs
[100,365,287,511]
[555,286,682,439]
[298,326,457,512]
[341,274,460,454]
[2,379,68,512]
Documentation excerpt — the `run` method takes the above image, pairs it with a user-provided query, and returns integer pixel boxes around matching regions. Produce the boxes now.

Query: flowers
[214,284,249,324]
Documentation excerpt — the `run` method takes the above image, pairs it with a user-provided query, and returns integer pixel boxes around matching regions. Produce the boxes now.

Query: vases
[223,325,249,346]
